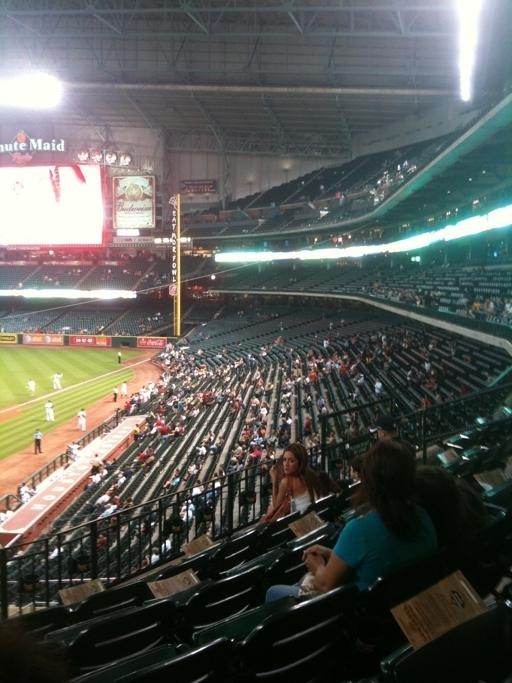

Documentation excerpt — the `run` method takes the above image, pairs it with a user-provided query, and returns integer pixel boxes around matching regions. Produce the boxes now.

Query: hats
[370,415,394,432]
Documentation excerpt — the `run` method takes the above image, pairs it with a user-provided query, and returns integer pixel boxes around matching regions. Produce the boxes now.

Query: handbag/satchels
[255,476,293,534]
[297,572,322,599]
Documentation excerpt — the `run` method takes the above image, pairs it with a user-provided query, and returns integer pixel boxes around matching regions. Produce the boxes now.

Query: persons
[1,147,510,620]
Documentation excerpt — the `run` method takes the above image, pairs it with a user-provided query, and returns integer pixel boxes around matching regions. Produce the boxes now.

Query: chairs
[2,414,511,682]
